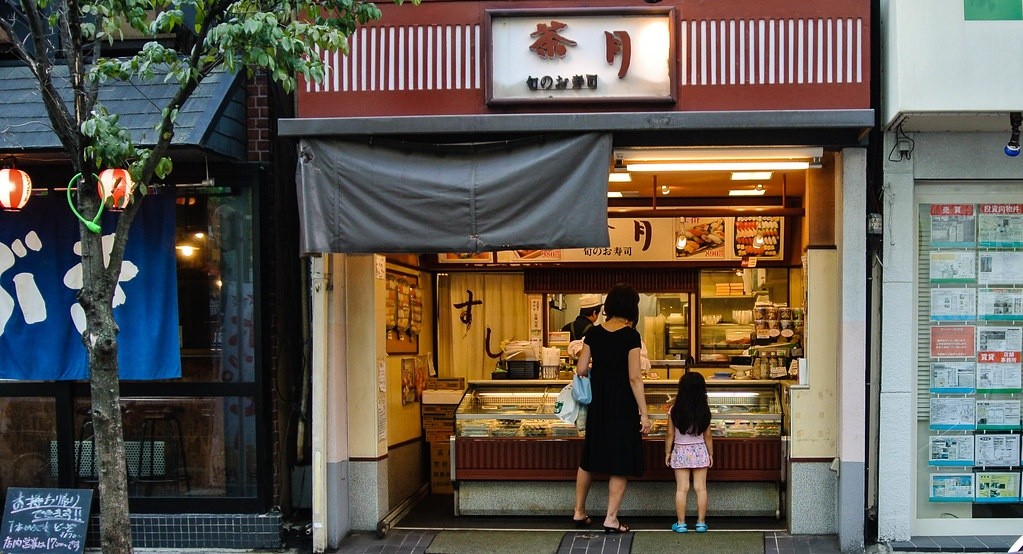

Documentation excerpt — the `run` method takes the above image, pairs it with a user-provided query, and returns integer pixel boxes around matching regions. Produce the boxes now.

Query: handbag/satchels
[576,405,588,430]
[554,382,579,424]
[571,372,592,406]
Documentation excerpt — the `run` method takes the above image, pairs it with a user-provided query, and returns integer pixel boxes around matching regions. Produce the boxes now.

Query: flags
[0,53,249,163]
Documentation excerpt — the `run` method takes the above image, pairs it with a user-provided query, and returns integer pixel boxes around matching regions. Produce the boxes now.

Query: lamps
[753,216,763,248]
[1004,112,1023,157]
[175,195,207,257]
[755,184,763,192]
[660,185,669,194]
[676,218,687,249]
[613,147,823,171]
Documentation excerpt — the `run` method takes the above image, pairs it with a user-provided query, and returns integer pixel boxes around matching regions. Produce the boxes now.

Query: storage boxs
[421,377,466,494]
[507,360,540,379]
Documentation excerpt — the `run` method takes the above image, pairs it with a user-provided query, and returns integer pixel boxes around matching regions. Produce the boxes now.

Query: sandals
[672,522,687,532]
[695,523,708,533]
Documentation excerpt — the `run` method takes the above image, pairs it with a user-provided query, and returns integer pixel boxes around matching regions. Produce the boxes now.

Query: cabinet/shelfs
[696,268,756,363]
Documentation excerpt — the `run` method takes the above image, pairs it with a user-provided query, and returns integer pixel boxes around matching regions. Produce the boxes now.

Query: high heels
[573,515,593,528]
[602,521,631,534]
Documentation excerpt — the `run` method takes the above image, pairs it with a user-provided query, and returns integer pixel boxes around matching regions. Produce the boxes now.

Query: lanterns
[0,169,31,212]
[98,169,135,212]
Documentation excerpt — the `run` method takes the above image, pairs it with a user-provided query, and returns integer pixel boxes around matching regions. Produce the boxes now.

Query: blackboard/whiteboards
[0,487,94,554]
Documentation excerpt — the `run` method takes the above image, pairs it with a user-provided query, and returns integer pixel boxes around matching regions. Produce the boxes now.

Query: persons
[665,371,713,533]
[561,294,602,343]
[573,283,650,531]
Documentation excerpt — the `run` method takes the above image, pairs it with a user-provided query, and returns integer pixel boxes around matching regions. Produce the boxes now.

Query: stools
[75,407,193,498]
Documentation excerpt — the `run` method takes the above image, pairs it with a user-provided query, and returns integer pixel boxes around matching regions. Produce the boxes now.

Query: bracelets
[639,408,648,416]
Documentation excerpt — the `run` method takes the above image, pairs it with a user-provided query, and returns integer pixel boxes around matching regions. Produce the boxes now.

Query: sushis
[682,220,725,252]
[736,220,778,256]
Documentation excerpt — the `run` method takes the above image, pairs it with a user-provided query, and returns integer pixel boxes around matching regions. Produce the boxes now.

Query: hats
[579,294,604,308]
